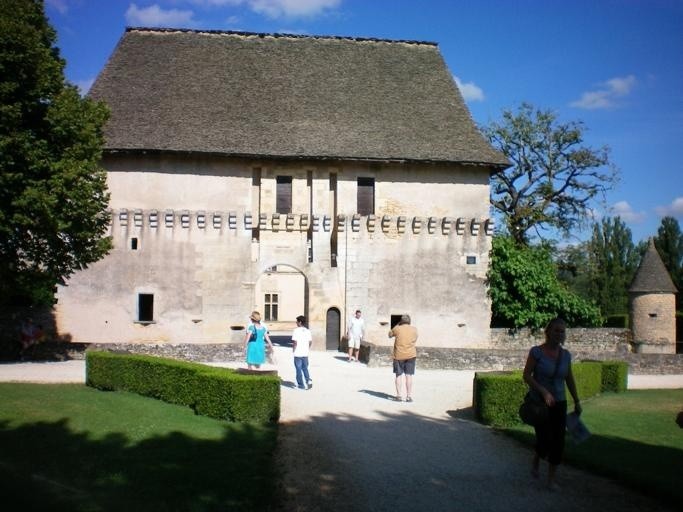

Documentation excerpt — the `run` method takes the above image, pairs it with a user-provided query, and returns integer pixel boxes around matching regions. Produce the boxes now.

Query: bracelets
[574,399,579,404]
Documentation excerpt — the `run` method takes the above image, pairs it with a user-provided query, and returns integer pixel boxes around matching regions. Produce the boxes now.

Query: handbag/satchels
[518,390,552,429]
[246,324,256,342]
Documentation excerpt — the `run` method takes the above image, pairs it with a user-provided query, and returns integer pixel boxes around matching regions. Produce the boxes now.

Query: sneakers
[307,380,311,389]
[405,397,412,402]
[393,397,401,401]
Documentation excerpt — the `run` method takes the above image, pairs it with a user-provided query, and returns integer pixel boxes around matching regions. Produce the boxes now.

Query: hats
[249,311,264,322]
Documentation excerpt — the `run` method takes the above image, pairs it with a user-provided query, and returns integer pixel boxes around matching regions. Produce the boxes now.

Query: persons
[388,314,418,402]
[523,318,582,491]
[291,316,312,390]
[243,311,274,369]
[347,309,366,363]
